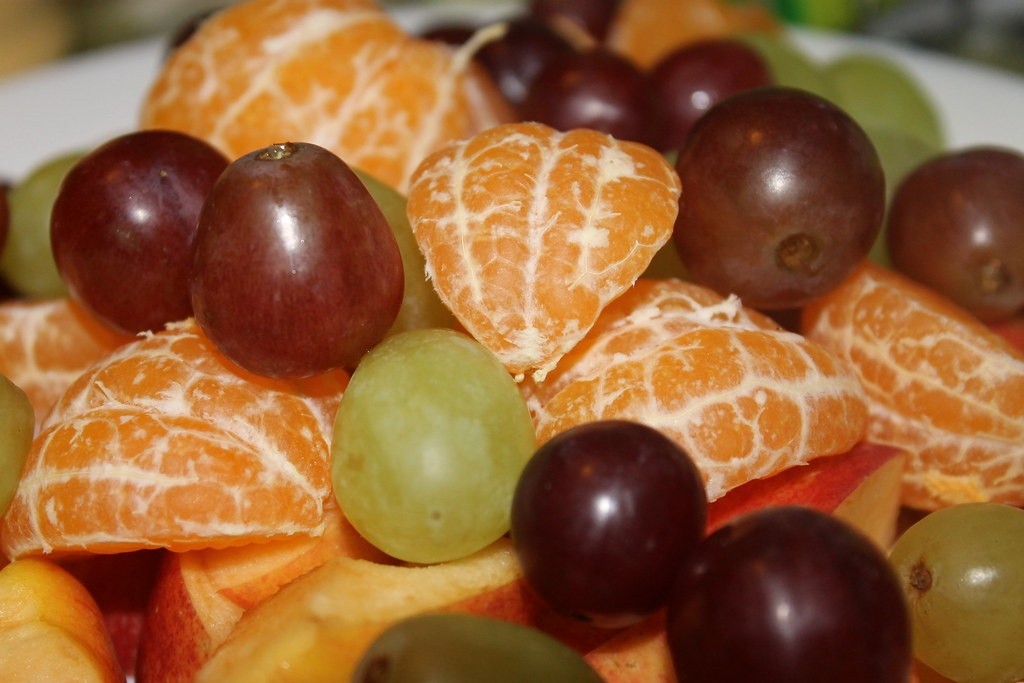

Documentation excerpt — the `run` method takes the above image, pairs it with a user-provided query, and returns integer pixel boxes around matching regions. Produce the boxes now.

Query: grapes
[0,24,1024,683]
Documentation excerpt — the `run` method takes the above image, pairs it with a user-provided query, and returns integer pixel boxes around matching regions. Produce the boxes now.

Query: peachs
[0,443,915,683]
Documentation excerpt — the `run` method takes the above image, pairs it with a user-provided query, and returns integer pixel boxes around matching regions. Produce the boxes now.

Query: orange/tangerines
[0,2,1024,563]
